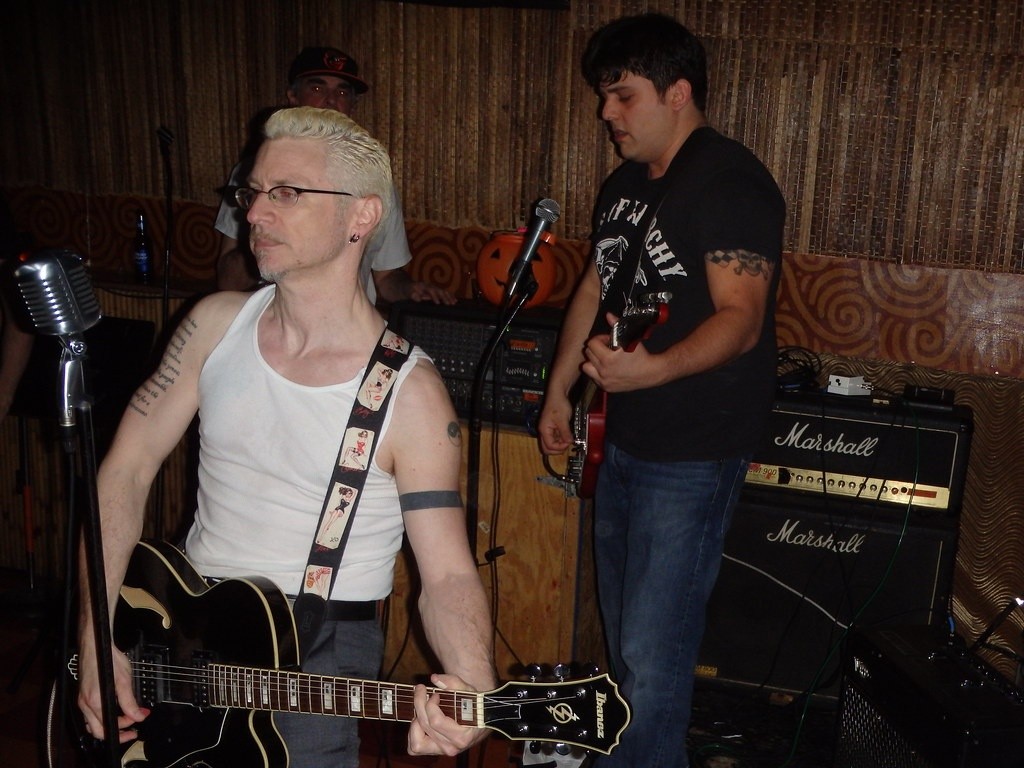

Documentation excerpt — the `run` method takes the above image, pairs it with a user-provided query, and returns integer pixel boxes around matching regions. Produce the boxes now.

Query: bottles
[134,215,152,287]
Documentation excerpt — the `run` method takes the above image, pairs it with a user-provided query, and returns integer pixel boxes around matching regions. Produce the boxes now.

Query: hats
[287,43,369,92]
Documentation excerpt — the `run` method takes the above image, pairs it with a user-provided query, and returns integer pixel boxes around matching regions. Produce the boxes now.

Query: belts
[281,596,387,624]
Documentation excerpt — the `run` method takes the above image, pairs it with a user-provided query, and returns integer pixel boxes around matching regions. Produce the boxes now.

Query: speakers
[693,487,959,701]
[833,624,1024,768]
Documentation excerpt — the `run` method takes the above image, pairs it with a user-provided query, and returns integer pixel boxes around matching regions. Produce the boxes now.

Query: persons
[77,105,500,768]
[214,47,458,327]
[540,12,786,768]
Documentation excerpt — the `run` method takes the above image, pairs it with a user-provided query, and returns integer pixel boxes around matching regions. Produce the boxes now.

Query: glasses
[233,183,354,210]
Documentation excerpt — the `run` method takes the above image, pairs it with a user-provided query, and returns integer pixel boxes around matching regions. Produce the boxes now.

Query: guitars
[559,288,676,503]
[43,535,635,768]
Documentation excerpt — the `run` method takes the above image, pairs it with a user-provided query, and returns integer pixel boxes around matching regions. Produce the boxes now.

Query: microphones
[504,198,560,301]
[16,251,102,335]
